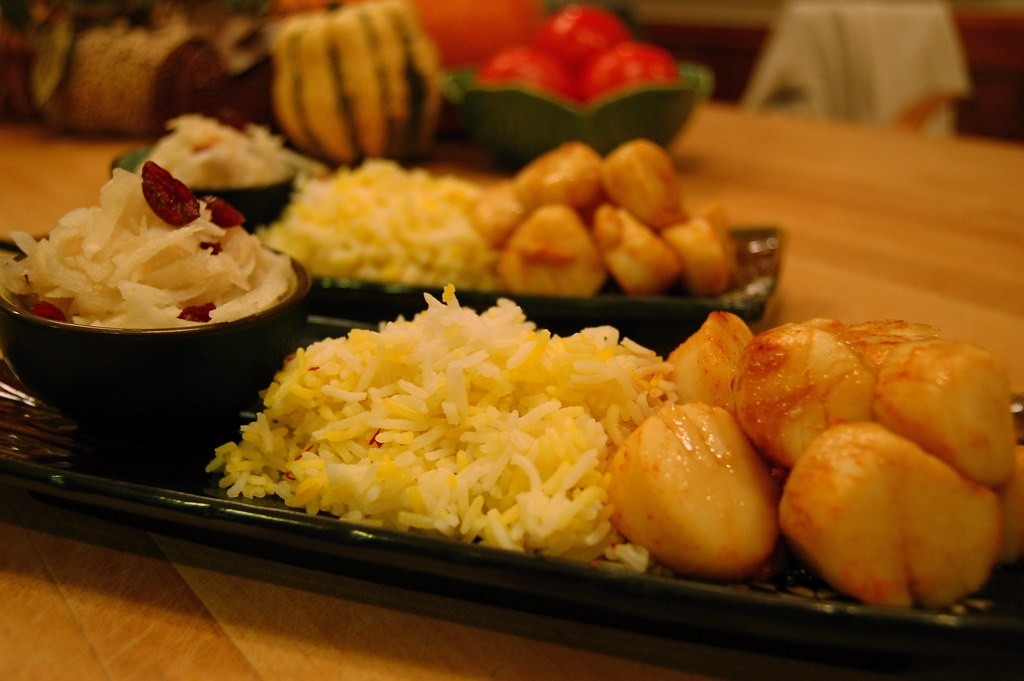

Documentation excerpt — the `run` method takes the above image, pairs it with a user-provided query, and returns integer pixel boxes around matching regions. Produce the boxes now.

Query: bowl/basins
[439,65,714,172]
[2,237,313,434]
[110,146,304,232]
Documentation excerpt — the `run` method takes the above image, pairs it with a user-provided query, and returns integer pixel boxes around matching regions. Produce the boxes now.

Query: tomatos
[415,0,680,112]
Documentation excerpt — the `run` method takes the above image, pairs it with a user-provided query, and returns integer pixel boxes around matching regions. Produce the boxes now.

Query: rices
[206,282,678,576]
[249,155,498,290]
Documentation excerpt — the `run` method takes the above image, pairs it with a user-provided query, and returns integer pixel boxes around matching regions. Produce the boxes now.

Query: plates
[0,317,1024,679]
[310,225,786,338]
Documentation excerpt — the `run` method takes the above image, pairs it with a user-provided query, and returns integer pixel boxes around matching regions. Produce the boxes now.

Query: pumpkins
[0,0,451,166]
